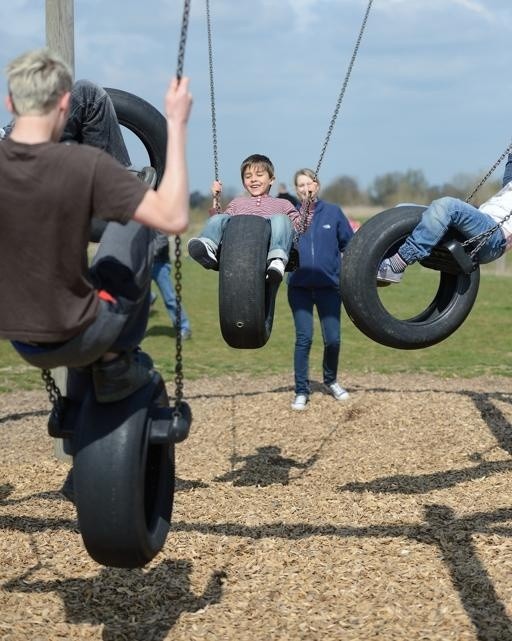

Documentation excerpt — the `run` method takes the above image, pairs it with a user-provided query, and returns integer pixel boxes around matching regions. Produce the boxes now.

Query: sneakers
[91,350,156,403]
[376,257,406,284]
[136,166,158,190]
[318,381,352,403]
[266,258,286,287]
[187,237,219,270]
[292,391,309,411]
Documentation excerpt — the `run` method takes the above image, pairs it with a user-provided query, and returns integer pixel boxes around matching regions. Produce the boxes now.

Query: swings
[42,0,190,442]
[207,0,372,272]
[418,144,512,276]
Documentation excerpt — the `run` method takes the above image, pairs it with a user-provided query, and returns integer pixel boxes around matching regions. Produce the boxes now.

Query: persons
[150,232,192,341]
[376,151,512,287]
[0,47,193,403]
[286,168,356,411]
[0,79,158,189]
[187,154,318,285]
